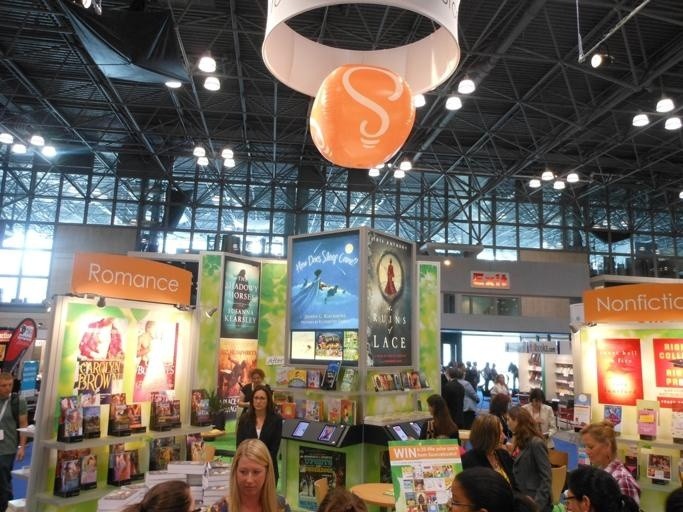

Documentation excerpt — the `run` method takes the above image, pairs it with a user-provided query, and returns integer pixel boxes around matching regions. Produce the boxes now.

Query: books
[410,421,421,435]
[95,460,233,511]
[318,424,336,441]
[603,404,683,482]
[394,426,407,441]
[56,387,210,491]
[292,421,309,436]
[275,362,432,424]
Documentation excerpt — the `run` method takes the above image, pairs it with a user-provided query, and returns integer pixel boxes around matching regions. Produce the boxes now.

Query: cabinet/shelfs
[271,383,435,446]
[8,404,214,510]
[613,433,683,491]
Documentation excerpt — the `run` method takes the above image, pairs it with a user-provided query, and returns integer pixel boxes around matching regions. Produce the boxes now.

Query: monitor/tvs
[317,424,337,442]
[392,425,410,441]
[409,423,422,437]
[290,420,311,437]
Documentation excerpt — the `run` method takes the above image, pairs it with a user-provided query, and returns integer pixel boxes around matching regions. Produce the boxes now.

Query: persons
[134,320,158,405]
[235,385,282,488]
[233,270,250,327]
[1,372,28,512]
[383,258,399,294]
[563,466,638,512]
[299,457,346,498]
[315,486,368,512]
[419,359,555,511]
[224,356,247,395]
[663,487,683,512]
[582,419,642,506]
[238,369,274,415]
[318,334,340,355]
[210,439,291,512]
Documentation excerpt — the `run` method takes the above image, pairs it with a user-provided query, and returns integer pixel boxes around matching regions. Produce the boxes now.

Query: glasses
[450,496,476,507]
[561,493,581,503]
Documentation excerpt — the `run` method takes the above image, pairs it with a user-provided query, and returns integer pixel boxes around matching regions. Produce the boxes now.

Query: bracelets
[18,445,24,448]
